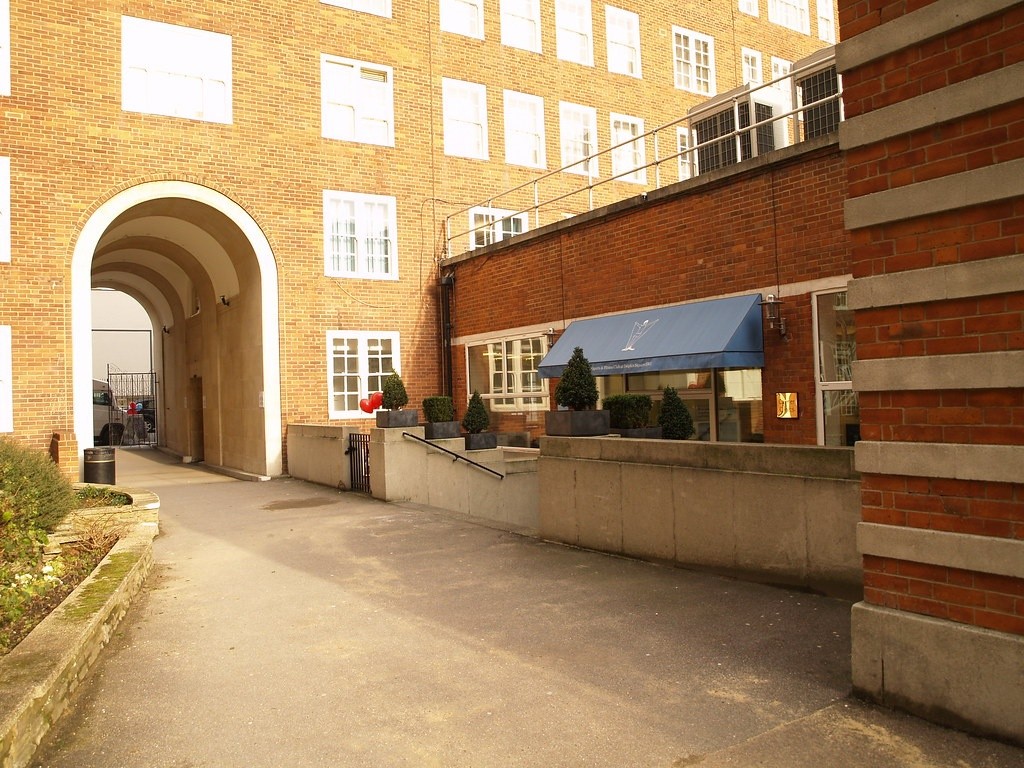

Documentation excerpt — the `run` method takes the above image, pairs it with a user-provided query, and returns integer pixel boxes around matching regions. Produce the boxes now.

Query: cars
[129,398,156,433]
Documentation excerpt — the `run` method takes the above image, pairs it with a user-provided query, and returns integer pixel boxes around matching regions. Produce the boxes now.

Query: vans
[93,379,144,446]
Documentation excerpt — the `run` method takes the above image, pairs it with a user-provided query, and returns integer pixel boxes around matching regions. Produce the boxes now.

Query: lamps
[758,294,790,343]
[542,328,558,348]
[220,295,230,306]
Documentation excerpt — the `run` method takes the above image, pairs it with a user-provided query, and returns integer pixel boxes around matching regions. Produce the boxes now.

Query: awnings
[537,295,764,377]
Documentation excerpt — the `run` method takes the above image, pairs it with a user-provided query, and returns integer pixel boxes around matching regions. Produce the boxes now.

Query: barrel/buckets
[83,448,115,485]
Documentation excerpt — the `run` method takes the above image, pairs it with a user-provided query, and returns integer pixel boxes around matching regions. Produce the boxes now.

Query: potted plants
[419,396,461,439]
[461,389,497,450]
[601,393,663,439]
[545,347,610,436]
[377,367,418,427]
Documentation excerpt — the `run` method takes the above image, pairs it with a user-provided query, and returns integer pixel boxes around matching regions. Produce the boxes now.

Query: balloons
[127,402,142,414]
[360,398,374,413]
[370,392,382,408]
